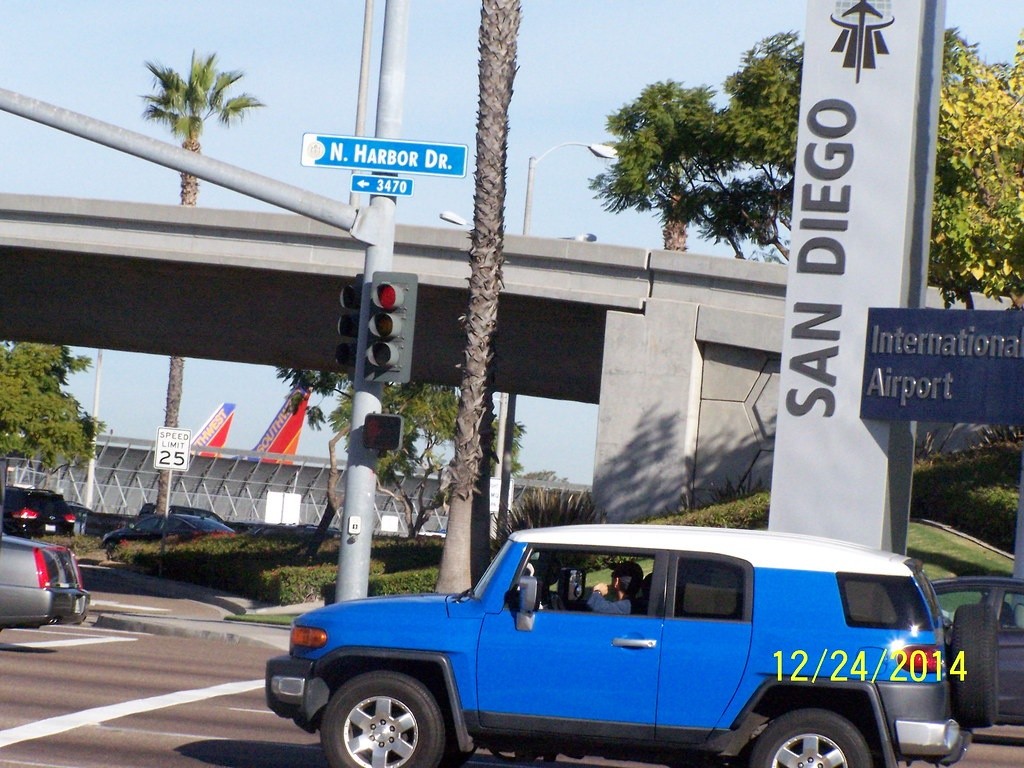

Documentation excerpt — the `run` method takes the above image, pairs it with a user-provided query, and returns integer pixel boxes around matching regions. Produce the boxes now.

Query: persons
[586,561,643,615]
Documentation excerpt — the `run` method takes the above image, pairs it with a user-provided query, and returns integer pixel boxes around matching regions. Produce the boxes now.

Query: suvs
[0,484,76,542]
[265,519,967,768]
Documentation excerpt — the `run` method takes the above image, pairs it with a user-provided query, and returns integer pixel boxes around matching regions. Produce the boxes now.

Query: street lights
[522,142,619,239]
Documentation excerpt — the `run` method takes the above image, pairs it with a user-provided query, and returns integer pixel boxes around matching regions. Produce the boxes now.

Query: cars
[139,501,224,533]
[100,510,239,562]
[925,576,1024,730]
[1,530,93,634]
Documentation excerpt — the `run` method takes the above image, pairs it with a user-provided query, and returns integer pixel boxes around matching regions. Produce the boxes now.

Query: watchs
[593,590,602,596]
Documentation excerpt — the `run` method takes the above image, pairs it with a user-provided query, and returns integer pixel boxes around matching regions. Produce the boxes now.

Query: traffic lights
[333,270,418,385]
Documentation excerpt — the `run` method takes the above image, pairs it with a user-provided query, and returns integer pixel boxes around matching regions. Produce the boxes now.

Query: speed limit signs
[153,426,192,471]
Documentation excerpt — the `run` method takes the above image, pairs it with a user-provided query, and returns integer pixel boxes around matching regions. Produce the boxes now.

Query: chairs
[631,573,653,616]
[1013,602,1024,628]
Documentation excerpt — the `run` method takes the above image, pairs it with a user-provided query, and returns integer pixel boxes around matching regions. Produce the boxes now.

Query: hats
[608,561,643,590]
